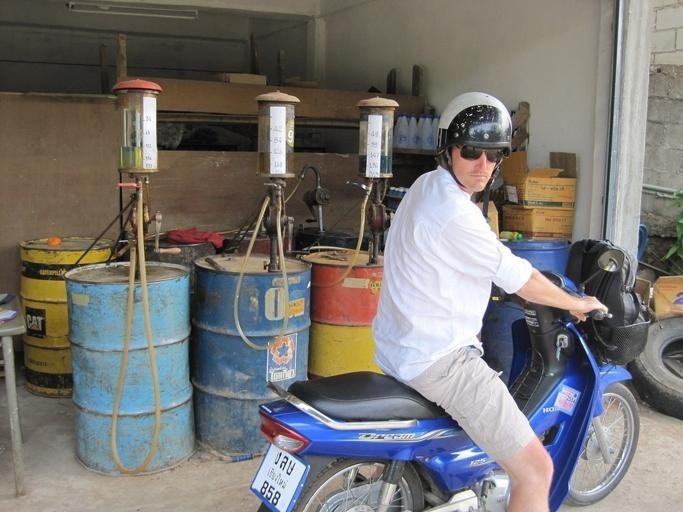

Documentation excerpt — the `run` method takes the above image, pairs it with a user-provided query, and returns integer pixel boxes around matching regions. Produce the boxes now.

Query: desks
[1,294,27,495]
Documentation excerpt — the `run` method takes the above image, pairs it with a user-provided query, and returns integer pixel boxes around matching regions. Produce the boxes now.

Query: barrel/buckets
[64,260,198,476]
[116,232,217,337]
[295,227,365,254]
[191,253,311,459]
[301,250,384,379]
[217,229,297,258]
[481,237,572,389]
[18,235,116,398]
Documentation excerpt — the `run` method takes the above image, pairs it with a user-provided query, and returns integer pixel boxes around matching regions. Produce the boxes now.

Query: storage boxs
[502,148,577,210]
[502,204,573,242]
[633,272,682,323]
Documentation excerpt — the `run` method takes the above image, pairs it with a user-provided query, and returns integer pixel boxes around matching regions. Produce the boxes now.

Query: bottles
[392,116,438,151]
[499,231,522,240]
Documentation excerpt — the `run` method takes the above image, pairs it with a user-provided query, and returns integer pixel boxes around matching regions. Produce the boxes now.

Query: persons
[372,91,608,512]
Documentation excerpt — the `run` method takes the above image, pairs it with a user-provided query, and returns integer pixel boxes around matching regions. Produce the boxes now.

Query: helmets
[433,91,514,171]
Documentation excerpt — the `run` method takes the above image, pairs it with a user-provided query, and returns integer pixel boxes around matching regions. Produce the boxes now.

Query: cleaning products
[393,115,440,152]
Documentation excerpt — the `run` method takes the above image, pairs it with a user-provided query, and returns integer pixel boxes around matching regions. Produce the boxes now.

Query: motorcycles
[247,246,649,511]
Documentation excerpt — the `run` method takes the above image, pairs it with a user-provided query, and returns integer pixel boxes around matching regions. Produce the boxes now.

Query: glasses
[454,144,504,163]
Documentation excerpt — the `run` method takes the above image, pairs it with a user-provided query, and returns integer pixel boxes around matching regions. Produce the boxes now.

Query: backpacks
[563,238,649,361]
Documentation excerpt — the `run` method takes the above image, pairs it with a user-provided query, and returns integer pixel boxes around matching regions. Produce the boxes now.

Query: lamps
[65,0,200,23]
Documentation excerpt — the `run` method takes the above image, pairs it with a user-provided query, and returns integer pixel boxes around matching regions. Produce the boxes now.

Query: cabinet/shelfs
[381,147,441,216]
